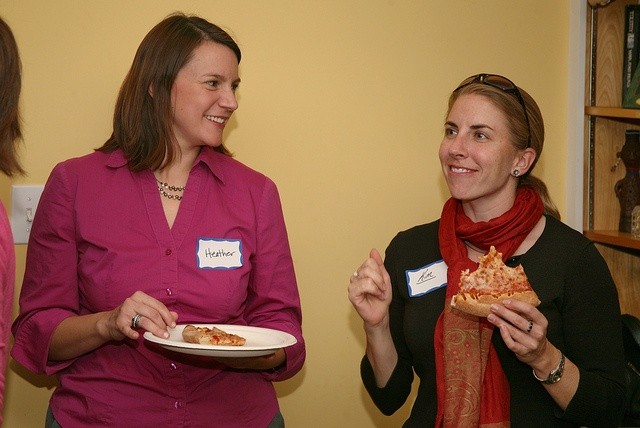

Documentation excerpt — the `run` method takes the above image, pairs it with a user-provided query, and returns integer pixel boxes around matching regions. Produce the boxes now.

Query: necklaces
[153,180,185,200]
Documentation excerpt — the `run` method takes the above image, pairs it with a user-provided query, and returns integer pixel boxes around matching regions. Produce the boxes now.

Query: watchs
[533,349,566,384]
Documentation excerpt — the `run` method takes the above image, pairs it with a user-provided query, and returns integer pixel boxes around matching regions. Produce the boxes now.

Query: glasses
[453,74,531,148]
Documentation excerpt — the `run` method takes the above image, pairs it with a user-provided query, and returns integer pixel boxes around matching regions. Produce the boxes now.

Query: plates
[142,323,299,358]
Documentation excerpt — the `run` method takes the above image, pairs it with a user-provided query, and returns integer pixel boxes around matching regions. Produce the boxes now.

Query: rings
[527,321,535,333]
[131,313,141,327]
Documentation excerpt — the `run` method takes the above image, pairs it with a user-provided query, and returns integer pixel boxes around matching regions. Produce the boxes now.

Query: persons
[11,13,305,427]
[348,74,627,428]
[0,14,27,427]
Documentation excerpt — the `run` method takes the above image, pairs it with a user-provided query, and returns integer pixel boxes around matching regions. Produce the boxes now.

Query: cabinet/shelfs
[582,1,640,325]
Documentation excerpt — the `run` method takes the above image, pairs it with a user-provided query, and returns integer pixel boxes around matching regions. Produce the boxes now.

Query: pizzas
[452,246,544,319]
[181,324,248,348]
